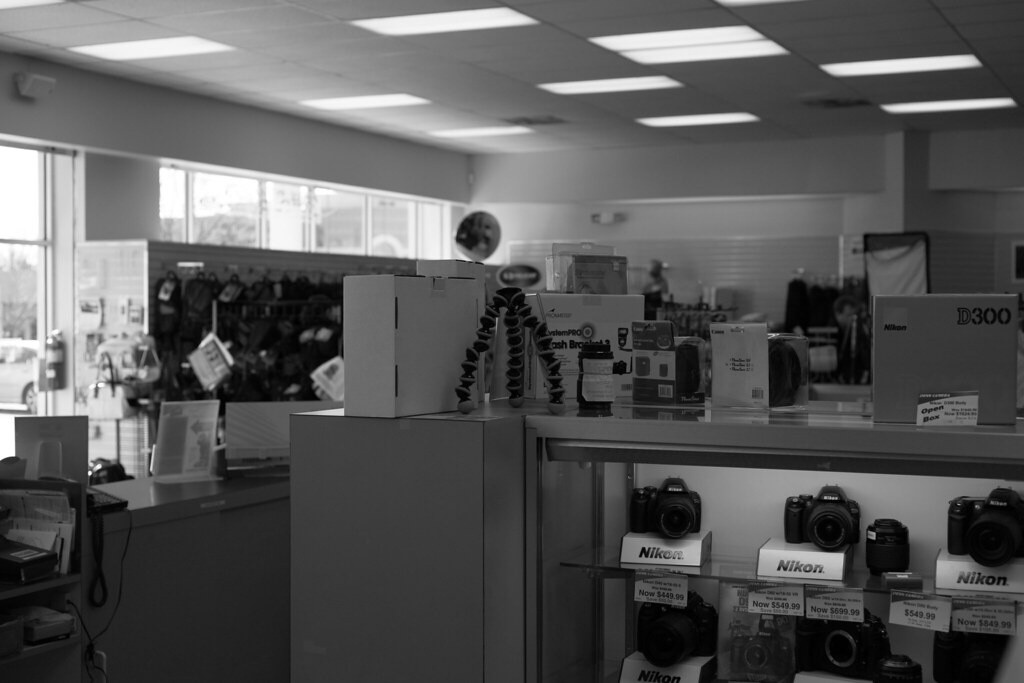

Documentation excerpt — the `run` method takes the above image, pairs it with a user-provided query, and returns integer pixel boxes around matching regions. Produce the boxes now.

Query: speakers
[17,72,56,97]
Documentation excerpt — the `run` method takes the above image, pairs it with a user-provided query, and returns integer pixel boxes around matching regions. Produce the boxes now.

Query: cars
[0,339,39,414]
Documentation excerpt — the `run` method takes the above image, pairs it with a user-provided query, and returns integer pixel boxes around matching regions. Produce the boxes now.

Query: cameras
[628,477,702,539]
[635,590,719,669]
[784,485,860,550]
[948,488,1024,566]
[795,608,891,680]
[730,628,791,677]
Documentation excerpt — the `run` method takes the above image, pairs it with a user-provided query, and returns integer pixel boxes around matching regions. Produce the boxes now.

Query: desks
[92,467,291,683]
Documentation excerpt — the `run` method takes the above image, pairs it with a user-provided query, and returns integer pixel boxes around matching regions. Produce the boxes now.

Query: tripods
[454,288,565,414]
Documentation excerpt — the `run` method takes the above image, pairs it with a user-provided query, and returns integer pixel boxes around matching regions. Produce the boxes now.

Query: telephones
[86,486,128,515]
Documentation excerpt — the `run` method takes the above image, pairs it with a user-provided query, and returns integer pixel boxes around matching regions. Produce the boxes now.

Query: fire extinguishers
[44,329,65,392]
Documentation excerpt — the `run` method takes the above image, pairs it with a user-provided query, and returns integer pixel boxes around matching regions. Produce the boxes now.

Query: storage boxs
[339,242,645,418]
[289,393,579,683]
[871,292,1019,427]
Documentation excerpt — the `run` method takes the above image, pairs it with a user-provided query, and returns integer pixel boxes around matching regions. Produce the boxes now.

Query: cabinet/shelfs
[527,420,1024,683]
[0,479,88,683]
